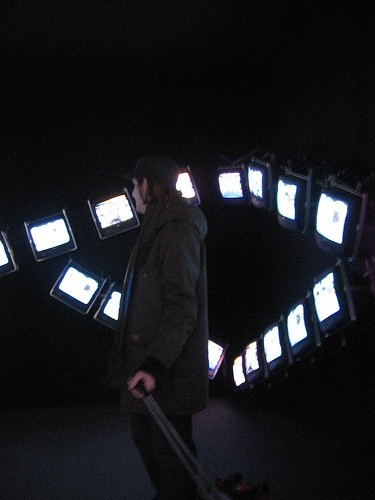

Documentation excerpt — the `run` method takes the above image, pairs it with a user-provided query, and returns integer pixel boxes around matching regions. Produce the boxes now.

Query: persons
[121,152,207,500]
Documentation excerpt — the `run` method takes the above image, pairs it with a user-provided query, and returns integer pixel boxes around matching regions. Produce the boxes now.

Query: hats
[121,155,179,184]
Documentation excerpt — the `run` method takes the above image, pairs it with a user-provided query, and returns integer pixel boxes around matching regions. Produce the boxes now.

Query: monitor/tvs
[0,159,358,394]
